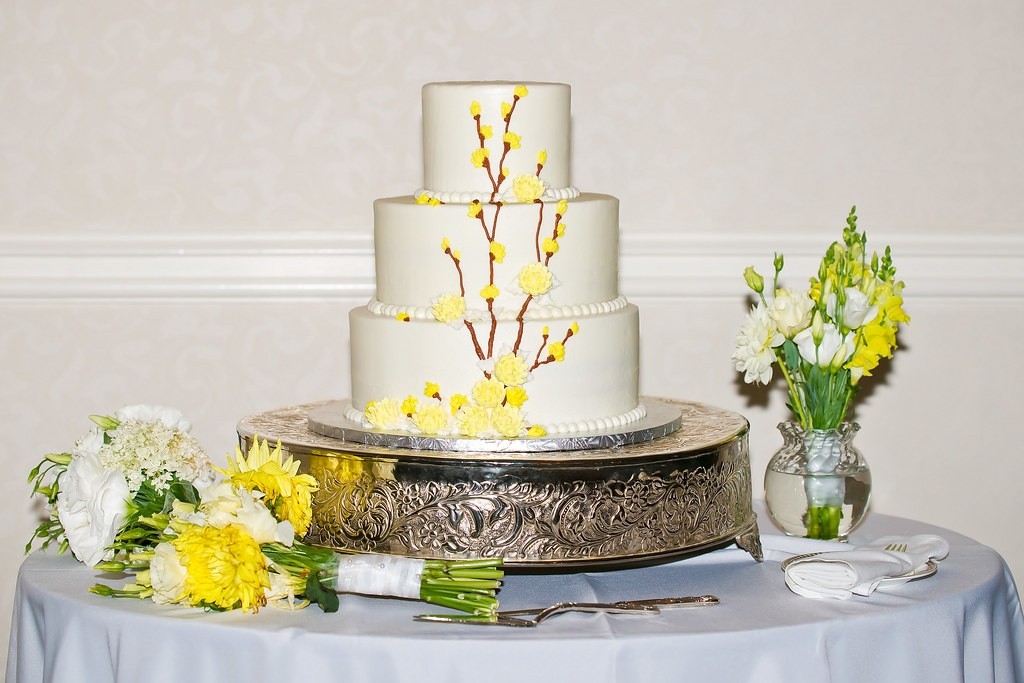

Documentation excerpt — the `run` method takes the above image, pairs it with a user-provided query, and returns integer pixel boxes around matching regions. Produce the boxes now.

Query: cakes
[350,83,645,432]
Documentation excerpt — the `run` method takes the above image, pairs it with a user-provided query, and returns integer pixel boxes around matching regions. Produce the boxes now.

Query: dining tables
[6,512,1023,681]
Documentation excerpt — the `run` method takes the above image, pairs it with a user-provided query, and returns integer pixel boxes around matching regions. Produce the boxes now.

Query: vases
[767,419,871,537]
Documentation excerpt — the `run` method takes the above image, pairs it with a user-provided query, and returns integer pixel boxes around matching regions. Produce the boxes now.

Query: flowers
[92,489,507,624]
[734,207,907,536]
[361,86,579,438]
[24,404,317,578]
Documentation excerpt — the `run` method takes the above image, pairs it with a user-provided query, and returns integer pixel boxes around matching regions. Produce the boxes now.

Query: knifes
[492,594,720,610]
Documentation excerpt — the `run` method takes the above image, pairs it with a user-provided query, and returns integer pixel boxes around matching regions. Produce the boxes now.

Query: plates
[782,552,937,588]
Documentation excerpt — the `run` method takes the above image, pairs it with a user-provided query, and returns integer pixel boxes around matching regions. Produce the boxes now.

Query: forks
[884,544,908,552]
